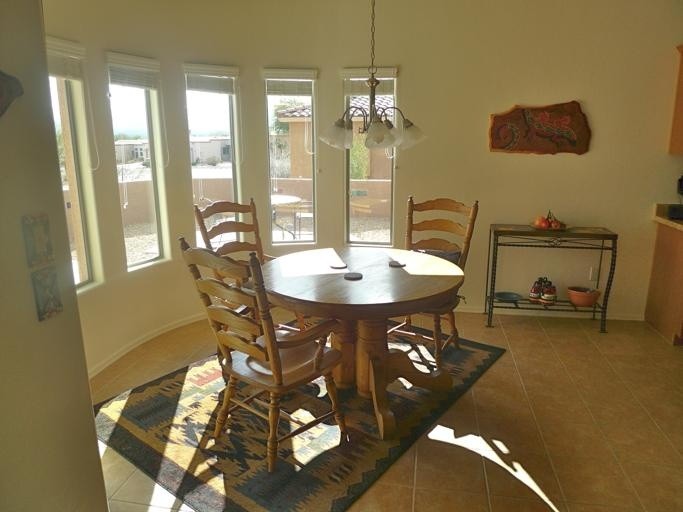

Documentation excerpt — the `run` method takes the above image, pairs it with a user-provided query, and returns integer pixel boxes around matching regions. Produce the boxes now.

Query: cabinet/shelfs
[664,44,682,160]
[643,202,683,347]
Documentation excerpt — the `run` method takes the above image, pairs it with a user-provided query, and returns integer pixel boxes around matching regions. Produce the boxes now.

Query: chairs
[197,197,235,246]
[296,201,313,239]
[178,238,349,471]
[384,194,479,370]
[192,199,313,369]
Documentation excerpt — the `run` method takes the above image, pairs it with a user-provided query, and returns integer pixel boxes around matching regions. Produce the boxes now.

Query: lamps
[315,1,425,155]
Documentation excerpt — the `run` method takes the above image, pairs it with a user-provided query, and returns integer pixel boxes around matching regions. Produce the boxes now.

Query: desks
[484,222,618,335]
[270,194,301,241]
[256,246,465,438]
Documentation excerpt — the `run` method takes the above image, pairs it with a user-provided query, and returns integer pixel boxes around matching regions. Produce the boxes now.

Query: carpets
[93,316,509,509]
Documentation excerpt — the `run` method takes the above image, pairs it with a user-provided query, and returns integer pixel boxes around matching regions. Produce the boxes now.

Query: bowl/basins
[567,286,599,307]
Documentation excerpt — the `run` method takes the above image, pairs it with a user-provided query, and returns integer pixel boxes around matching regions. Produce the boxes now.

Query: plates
[528,222,575,232]
[493,292,522,301]
